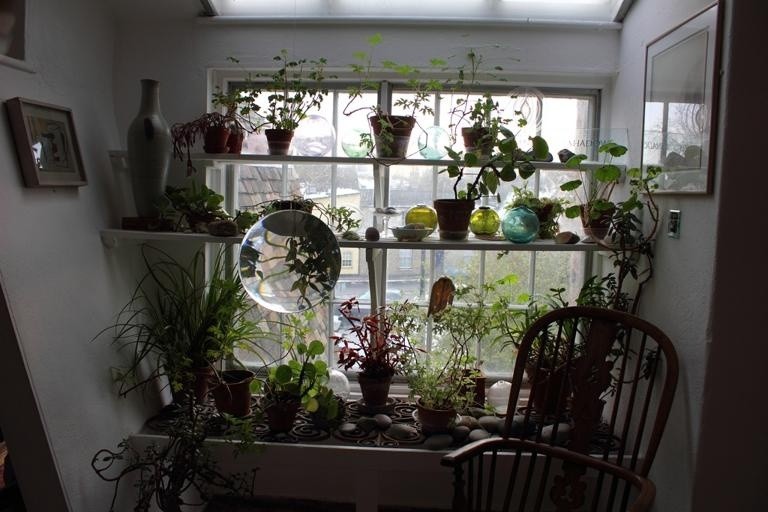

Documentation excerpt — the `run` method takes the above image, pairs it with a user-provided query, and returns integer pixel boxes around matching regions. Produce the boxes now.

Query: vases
[208,370,257,419]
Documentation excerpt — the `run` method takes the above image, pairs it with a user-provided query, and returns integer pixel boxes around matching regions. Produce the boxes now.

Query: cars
[356,288,403,301]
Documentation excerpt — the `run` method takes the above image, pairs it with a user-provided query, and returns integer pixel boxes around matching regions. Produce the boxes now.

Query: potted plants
[552,164,664,428]
[343,34,449,166]
[498,290,579,419]
[173,113,243,176]
[212,49,338,155]
[241,196,360,234]
[91,243,305,410]
[558,142,628,242]
[249,340,339,437]
[506,183,570,240]
[389,282,507,437]
[436,48,528,163]
[435,126,550,239]
[166,185,235,234]
[330,297,428,409]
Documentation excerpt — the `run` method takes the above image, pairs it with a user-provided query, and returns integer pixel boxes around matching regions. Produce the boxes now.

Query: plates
[392,226,435,239]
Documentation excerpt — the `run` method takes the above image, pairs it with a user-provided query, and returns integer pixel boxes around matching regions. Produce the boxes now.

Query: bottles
[126,77,176,218]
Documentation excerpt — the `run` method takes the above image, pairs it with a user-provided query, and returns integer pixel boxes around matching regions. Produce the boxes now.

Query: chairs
[437,306,680,511]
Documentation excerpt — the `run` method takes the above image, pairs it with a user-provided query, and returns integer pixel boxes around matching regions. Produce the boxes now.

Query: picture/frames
[5,96,89,189]
[640,0,725,197]
[0,1,38,75]
